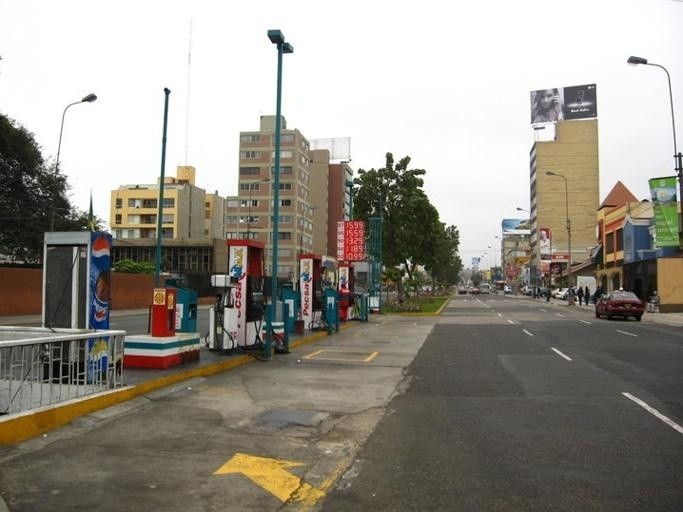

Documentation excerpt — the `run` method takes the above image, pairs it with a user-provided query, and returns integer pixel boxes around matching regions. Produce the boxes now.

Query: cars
[458,284,577,301]
[595,288,644,322]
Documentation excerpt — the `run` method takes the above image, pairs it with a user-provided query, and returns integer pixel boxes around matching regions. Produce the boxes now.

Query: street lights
[264,28,294,323]
[49,93,96,230]
[345,178,355,218]
[298,205,319,254]
[243,177,271,236]
[516,207,529,215]
[626,55,682,253]
[480,235,501,283]
[545,172,571,298]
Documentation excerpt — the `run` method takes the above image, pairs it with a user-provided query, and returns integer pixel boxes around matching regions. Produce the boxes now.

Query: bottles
[89,269,110,350]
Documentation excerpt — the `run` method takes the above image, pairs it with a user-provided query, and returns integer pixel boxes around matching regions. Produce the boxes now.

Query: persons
[530,87,563,123]
[503,284,602,306]
[538,230,549,253]
[645,290,659,312]
